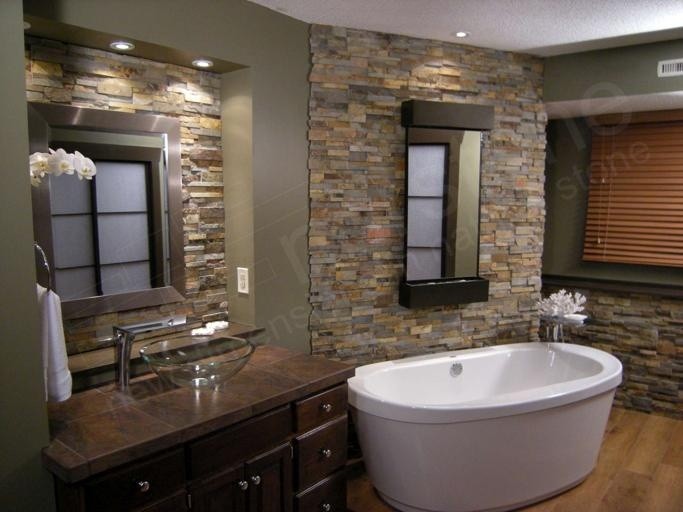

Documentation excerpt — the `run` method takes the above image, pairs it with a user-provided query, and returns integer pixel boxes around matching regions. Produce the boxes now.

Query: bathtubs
[347,342,623,506]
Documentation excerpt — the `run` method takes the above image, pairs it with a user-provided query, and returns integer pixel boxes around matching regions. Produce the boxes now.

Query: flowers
[28,145,97,188]
[533,288,586,319]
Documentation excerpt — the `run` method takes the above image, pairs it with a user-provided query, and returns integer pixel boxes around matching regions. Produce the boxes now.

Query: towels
[36,283,74,403]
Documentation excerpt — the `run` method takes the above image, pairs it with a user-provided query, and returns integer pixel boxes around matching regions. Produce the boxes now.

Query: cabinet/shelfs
[44,338,354,512]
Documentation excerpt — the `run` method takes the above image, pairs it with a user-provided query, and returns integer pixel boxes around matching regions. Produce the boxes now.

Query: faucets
[113,315,187,387]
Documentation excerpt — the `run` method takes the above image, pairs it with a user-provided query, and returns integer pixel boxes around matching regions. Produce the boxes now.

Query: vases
[540,319,566,343]
